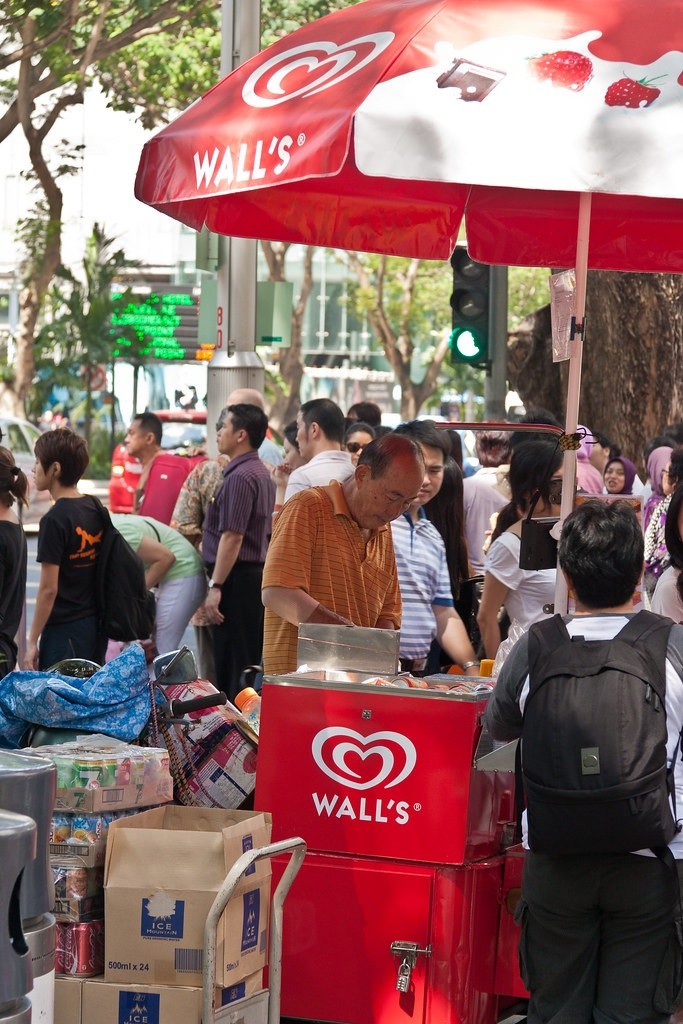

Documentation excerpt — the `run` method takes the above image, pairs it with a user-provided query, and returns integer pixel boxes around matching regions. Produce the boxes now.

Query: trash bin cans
[0,809,39,1024]
[1,747,57,1024]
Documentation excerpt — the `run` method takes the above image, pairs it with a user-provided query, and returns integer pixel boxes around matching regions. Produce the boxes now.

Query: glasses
[346,442,369,453]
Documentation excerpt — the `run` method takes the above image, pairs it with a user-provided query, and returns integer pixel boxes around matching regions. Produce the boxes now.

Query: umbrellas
[135,0,683,618]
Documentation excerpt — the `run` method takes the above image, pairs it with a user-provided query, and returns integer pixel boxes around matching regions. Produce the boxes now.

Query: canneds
[17,742,170,977]
[361,677,497,693]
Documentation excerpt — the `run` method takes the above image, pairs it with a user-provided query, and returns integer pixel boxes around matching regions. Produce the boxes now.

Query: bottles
[234,686,261,737]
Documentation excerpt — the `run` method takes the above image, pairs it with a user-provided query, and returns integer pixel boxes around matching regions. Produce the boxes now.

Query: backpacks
[513,609,683,852]
[86,495,155,640]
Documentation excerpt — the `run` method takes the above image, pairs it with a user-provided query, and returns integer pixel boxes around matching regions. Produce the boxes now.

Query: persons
[483,499,683,1024]
[22,385,393,700]
[0,445,29,681]
[260,411,683,684]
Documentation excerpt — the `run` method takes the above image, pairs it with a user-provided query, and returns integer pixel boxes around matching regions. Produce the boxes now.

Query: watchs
[208,579,222,589]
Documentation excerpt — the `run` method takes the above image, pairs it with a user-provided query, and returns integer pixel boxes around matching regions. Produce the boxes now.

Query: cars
[0,416,56,528]
[108,408,286,515]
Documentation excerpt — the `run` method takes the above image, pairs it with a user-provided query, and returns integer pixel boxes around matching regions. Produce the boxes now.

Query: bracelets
[462,660,480,670]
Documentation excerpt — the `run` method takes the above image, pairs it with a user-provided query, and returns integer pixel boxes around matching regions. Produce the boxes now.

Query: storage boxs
[268,844,500,1024]
[492,840,536,1000]
[48,777,274,1024]
[254,663,520,864]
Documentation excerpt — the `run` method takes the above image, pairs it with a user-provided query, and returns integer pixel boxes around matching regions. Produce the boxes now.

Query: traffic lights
[450,240,493,365]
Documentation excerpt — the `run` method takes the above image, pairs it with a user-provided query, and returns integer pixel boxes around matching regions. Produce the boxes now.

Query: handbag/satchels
[519,517,562,571]
